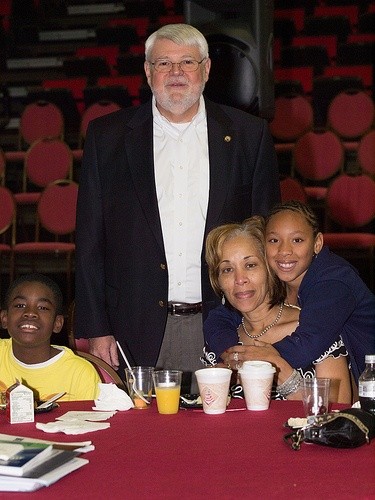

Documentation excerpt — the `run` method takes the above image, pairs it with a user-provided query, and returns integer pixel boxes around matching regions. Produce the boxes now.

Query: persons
[0,275,107,404]
[204,200,375,383]
[74,24,282,397]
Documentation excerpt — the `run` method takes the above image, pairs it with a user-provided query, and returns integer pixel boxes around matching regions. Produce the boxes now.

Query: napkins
[36,383,135,435]
[287,400,362,429]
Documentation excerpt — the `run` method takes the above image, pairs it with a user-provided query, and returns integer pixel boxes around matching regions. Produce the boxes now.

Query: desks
[0,393,375,500]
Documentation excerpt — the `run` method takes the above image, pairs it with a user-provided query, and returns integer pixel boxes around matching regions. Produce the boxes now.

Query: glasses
[149,57,206,73]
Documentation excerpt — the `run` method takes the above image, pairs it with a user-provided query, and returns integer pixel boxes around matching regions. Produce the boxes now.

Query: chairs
[0,0,375,309]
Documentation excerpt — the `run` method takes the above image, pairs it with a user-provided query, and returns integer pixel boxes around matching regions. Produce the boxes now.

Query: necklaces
[240,301,287,336]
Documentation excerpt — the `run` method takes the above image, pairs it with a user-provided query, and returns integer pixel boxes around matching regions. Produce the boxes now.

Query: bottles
[358,355,375,415]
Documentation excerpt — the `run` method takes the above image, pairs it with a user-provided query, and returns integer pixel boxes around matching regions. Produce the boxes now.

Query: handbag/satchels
[284,407,375,451]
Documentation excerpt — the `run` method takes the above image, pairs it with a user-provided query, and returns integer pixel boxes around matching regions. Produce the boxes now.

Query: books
[0,440,79,491]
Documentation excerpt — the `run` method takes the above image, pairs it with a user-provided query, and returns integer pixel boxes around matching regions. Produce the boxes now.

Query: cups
[299,377,330,416]
[125,366,154,410]
[194,368,232,414]
[152,370,182,415]
[238,361,277,411]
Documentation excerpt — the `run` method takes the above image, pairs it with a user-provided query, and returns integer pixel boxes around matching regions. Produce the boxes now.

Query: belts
[168,303,202,315]
